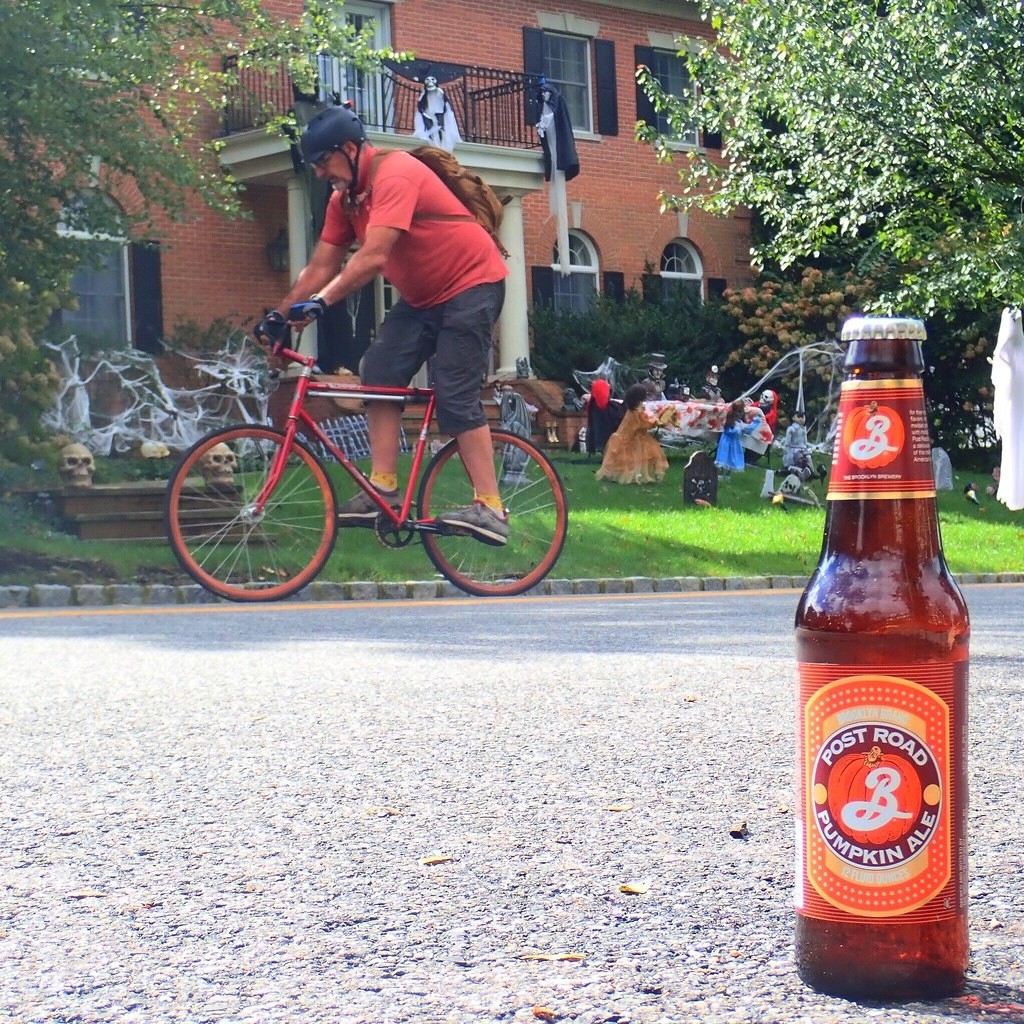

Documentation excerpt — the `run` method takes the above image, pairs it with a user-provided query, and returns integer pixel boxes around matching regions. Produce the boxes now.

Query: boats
[573,354,762,443]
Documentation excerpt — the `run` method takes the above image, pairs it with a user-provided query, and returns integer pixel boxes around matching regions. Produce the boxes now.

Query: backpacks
[340,145,513,261]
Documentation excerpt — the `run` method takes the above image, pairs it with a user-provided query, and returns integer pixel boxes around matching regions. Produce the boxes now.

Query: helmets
[300,106,367,163]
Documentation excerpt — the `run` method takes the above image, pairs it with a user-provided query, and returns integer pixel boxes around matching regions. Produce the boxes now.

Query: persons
[413,76,460,150]
[586,353,816,486]
[254,106,509,547]
[535,84,579,180]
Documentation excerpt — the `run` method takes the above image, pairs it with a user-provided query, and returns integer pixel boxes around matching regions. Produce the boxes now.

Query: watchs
[310,293,329,314]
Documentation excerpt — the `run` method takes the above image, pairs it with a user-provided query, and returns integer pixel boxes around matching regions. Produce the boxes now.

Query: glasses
[309,147,334,169]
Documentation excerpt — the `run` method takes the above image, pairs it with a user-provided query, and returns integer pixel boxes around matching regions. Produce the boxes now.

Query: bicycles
[163,312,570,603]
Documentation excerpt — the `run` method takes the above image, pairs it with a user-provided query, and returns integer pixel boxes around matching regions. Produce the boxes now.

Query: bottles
[795,316,970,1003]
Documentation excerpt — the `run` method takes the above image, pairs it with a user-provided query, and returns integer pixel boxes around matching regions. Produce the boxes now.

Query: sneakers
[338,486,403,517]
[436,503,509,546]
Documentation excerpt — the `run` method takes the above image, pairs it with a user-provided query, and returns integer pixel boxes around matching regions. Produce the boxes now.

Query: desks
[614,399,771,460]
